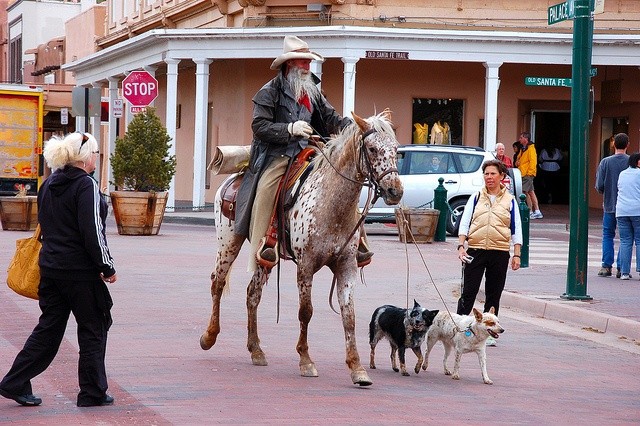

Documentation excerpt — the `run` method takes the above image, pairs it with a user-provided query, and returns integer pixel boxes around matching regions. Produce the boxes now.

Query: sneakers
[598,267,612,276]
[616,270,621,278]
[621,275,629,280]
[77,395,114,407]
[635,272,640,279]
[530,212,543,220]
[0,388,42,406]
[485,336,497,345]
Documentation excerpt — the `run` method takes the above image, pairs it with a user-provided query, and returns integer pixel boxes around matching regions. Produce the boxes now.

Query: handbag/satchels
[6,223,42,300]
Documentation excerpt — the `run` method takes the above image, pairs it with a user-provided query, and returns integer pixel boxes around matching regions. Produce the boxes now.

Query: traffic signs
[548,0,573,25]
[525,77,571,87]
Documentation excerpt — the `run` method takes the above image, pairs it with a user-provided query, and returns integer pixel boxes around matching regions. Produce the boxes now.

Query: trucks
[0,84,44,194]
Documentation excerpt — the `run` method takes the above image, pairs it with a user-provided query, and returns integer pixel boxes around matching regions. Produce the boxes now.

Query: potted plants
[108,107,177,236]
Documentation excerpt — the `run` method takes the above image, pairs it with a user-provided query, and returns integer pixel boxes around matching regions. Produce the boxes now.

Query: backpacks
[454,215,473,241]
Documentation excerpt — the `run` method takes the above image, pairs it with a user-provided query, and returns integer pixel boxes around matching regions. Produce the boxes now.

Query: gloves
[288,121,313,138]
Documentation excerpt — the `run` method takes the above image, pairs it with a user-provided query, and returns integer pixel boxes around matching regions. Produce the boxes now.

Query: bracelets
[513,255,521,258]
[457,245,464,251]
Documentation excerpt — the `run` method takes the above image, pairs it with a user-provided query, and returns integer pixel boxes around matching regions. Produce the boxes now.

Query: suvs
[358,145,523,236]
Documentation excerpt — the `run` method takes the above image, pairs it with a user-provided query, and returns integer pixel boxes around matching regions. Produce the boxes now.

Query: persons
[234,35,375,274]
[495,142,513,168]
[414,114,430,143]
[423,155,444,173]
[538,139,563,202]
[514,131,544,219]
[456,159,524,348]
[596,132,633,278]
[511,141,524,169]
[431,114,453,145]
[616,152,639,280]
[0,131,116,409]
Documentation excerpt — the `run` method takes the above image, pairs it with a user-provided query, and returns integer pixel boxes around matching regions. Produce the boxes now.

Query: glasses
[76,130,89,155]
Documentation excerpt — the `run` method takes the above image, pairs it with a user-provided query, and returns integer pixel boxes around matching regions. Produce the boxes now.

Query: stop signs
[122,71,158,107]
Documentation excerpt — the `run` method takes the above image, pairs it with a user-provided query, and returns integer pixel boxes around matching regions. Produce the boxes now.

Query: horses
[200,103,404,387]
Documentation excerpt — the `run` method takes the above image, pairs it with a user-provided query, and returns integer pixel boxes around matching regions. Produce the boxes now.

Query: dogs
[422,307,505,385]
[369,299,439,376]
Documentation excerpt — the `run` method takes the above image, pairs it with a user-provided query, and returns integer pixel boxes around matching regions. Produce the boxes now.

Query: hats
[269,35,325,70]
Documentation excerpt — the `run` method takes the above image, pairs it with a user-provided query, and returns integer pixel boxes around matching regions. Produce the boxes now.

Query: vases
[0,196,40,230]
[394,207,440,243]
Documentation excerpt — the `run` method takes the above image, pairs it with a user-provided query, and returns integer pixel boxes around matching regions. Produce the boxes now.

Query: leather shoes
[356,250,374,262]
[261,248,276,274]
